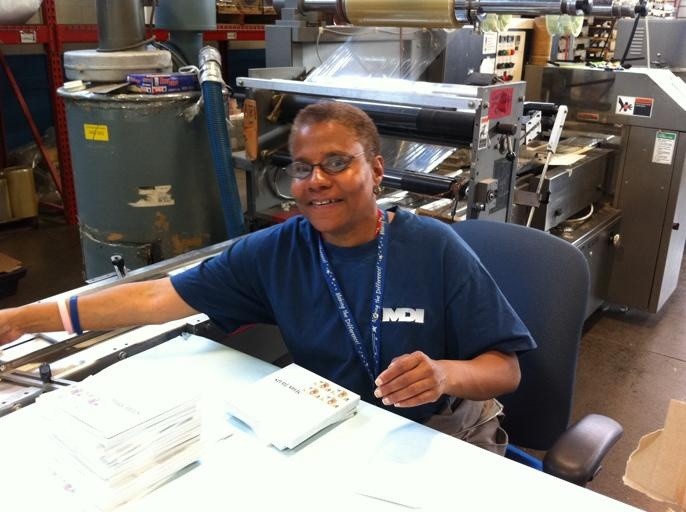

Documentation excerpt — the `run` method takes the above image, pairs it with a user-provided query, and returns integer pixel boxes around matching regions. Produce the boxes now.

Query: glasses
[279,147,377,180]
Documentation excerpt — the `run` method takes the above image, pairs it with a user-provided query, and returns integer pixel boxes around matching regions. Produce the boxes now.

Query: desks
[0,334,647,512]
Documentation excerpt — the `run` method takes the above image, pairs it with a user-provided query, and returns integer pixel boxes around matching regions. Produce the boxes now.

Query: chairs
[226,221,624,487]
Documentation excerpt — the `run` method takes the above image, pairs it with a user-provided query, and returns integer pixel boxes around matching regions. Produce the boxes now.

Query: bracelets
[70,294,82,336]
[58,300,76,335]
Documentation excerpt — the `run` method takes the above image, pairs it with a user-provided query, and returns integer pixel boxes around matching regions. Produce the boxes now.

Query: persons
[1,100,537,457]
